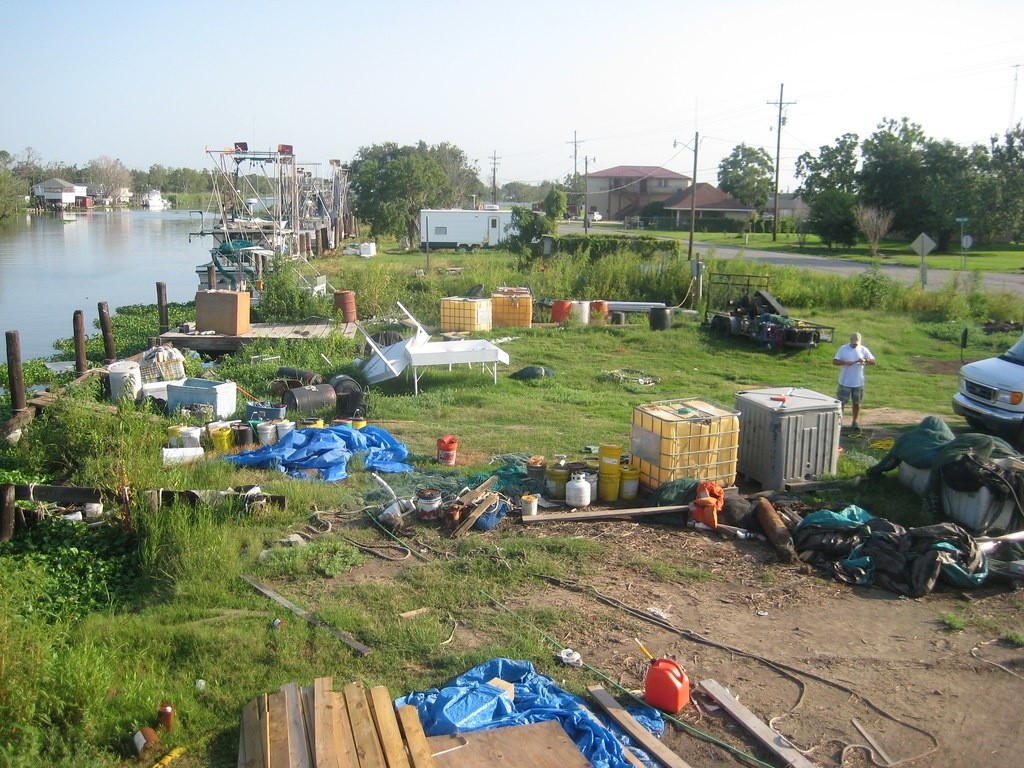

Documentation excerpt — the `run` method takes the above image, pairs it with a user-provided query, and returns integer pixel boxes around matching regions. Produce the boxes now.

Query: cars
[588,211,602,222]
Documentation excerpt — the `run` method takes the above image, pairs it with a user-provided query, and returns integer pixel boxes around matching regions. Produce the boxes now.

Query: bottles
[645,657,690,715]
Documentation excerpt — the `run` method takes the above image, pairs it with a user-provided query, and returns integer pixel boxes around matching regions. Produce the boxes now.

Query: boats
[194,240,336,305]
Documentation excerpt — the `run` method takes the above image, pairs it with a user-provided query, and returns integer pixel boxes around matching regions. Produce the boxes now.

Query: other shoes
[851,421,858,429]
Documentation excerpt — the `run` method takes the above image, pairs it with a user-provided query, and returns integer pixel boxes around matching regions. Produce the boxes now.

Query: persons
[833,332,876,429]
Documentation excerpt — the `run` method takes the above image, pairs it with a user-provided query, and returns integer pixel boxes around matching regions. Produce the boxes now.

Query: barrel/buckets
[416,487,443,521]
[133,726,161,759]
[347,389,370,416]
[446,508,460,529]
[435,439,458,466]
[107,361,142,404]
[282,384,337,413]
[650,306,671,331]
[276,367,324,386]
[552,299,609,324]
[328,374,362,407]
[85,503,104,518]
[527,442,641,502]
[334,289,357,323]
[166,418,368,449]
[612,311,625,324]
[157,702,175,732]
[521,494,538,516]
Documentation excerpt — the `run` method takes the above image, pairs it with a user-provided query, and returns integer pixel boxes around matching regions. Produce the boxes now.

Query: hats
[850,332,862,344]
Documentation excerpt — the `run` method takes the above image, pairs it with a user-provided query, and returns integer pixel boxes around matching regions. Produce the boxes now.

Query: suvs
[951,328,1024,452]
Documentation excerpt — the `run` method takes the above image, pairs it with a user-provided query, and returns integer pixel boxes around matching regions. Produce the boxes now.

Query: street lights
[672,140,697,259]
[571,153,588,237]
